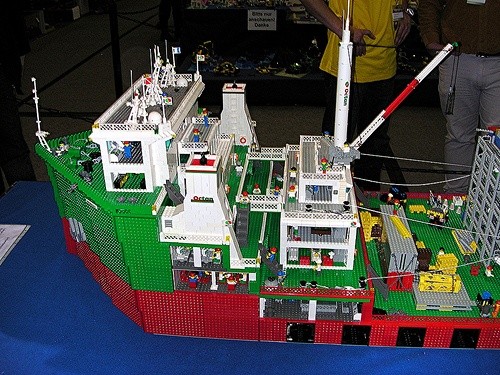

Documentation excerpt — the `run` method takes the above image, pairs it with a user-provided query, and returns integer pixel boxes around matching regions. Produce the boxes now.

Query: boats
[32,0,500,351]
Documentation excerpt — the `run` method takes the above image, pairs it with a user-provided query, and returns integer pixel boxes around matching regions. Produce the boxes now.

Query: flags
[162,47,205,105]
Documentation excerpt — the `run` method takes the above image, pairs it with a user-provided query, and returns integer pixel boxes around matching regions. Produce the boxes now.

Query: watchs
[403,8,414,18]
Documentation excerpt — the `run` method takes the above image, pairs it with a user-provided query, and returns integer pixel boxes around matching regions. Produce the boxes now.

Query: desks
[0,179,500,375]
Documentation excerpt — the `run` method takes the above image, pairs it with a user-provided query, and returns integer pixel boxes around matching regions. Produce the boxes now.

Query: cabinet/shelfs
[175,0,441,107]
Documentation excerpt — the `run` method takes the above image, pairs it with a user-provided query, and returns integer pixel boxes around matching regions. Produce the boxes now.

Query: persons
[301,0,419,191]
[272,174,282,196]
[394,200,400,215]
[266,247,319,289]
[320,158,330,174]
[288,167,297,203]
[418,0,500,193]
[486,266,492,276]
[359,277,366,289]
[159,0,185,41]
[480,292,492,317]
[439,247,444,254]
[178,247,237,289]
[193,111,208,141]
[122,141,134,161]
[0,0,56,198]
[235,160,261,204]
[59,141,65,154]
